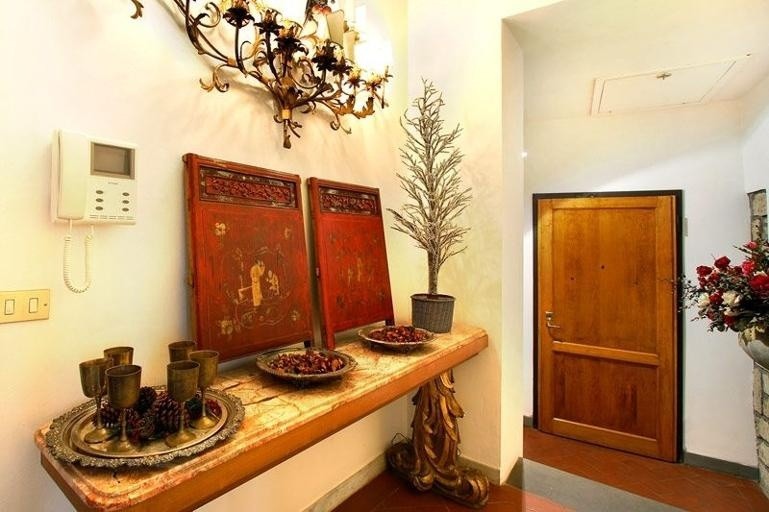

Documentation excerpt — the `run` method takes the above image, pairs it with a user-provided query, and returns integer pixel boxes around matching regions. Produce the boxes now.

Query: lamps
[131,0,393,148]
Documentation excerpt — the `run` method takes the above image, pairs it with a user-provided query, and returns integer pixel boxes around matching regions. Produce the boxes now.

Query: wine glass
[78,339,221,454]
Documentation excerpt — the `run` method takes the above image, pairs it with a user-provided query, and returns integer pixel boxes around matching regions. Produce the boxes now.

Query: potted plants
[387,77,472,333]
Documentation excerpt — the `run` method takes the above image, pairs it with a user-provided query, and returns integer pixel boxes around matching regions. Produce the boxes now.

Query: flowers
[662,237,769,345]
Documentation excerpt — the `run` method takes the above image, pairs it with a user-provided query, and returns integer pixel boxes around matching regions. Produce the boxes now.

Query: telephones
[51,129,140,226]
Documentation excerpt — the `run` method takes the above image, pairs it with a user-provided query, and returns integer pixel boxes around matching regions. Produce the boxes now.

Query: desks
[36,321,488,512]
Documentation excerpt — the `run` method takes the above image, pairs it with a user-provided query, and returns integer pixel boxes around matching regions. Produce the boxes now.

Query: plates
[253,346,358,385]
[358,324,438,354]
